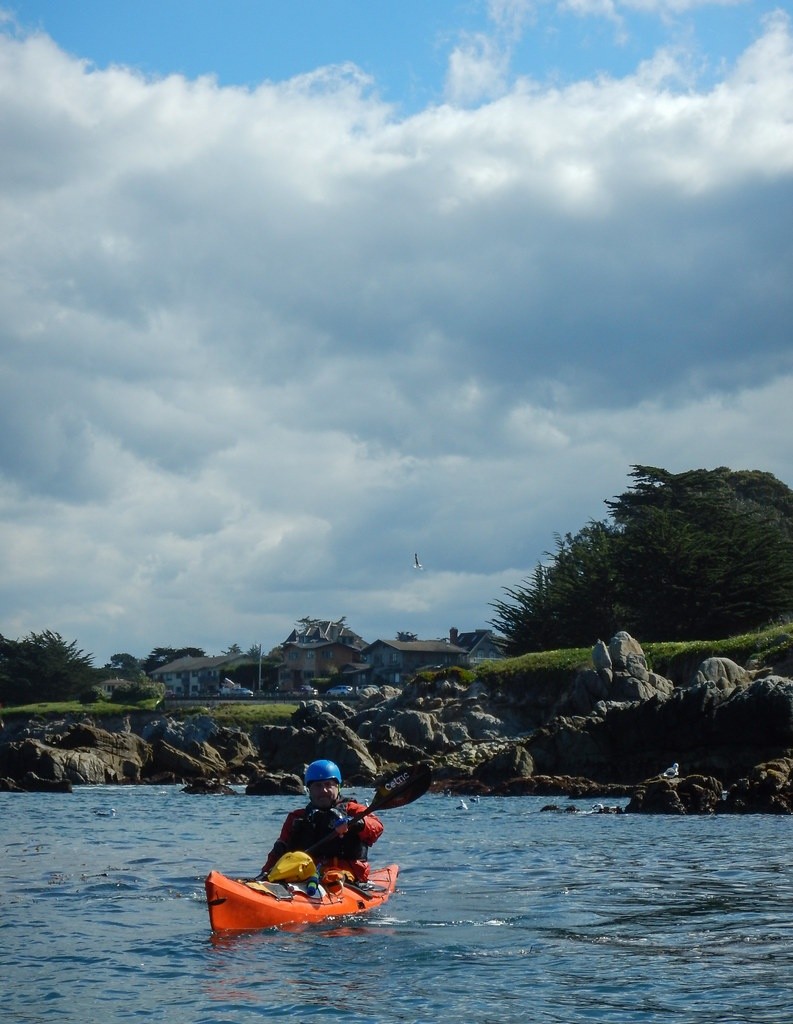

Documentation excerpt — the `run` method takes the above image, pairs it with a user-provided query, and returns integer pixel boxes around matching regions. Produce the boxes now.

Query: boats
[203,863,401,935]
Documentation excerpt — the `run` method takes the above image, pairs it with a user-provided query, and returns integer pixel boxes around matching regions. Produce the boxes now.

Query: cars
[297,685,314,694]
[216,684,254,698]
[326,686,353,695]
[356,685,380,695]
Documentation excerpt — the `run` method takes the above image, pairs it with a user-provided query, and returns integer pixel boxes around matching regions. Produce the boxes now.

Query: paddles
[256,761,435,880]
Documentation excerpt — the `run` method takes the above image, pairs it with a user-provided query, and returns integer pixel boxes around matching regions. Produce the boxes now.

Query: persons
[260,759,383,884]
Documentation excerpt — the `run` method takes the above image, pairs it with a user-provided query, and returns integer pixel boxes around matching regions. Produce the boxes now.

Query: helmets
[305,760,342,788]
